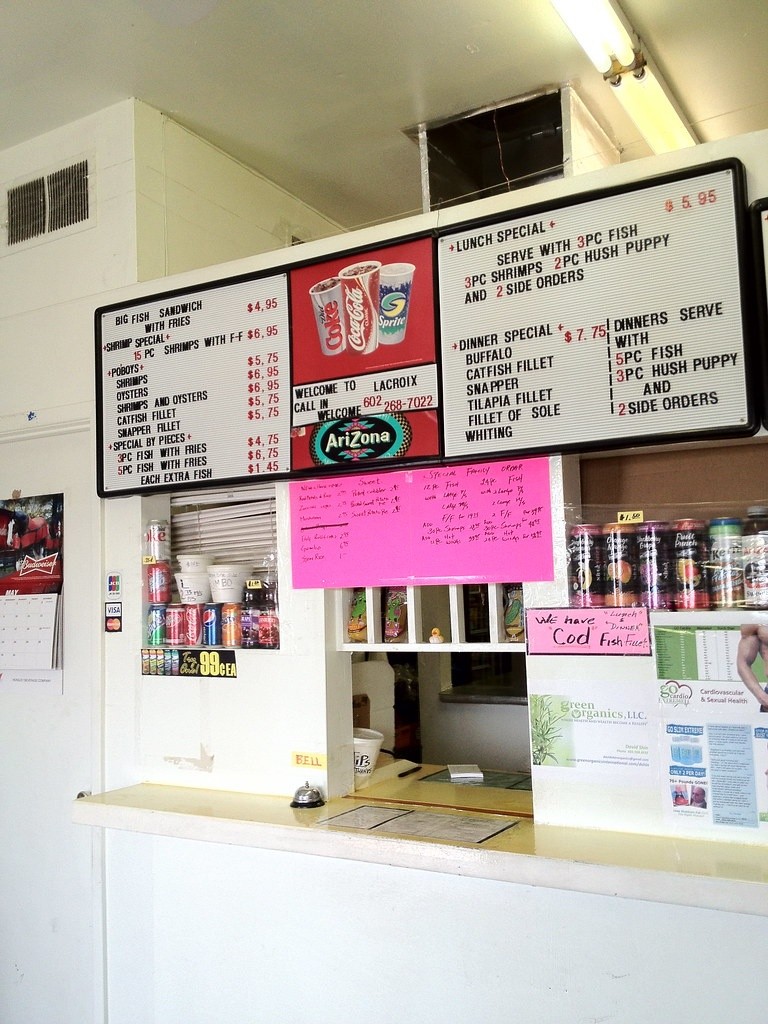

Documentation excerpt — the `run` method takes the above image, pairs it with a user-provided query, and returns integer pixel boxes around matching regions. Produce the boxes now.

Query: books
[448,764,484,778]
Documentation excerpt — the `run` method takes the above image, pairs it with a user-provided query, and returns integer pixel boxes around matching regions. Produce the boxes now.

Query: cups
[338,260,382,355]
[308,276,347,355]
[379,263,416,345]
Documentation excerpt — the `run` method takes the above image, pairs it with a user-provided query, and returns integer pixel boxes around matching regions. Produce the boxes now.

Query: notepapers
[618,510,644,525]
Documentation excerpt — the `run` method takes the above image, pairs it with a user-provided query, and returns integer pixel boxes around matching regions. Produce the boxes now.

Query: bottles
[141,649,179,676]
[264,560,277,604]
[242,576,265,606]
[742,506,768,610]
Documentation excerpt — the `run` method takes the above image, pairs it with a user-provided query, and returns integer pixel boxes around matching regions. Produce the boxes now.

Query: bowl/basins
[174,573,211,604]
[353,727,384,777]
[206,564,251,602]
[177,554,214,574]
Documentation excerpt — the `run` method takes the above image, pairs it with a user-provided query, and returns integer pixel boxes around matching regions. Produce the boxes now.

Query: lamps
[550,0,647,89]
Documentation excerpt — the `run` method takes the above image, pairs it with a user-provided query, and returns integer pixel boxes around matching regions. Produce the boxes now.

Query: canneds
[147,561,172,605]
[571,515,745,612]
[145,518,172,564]
[147,601,242,647]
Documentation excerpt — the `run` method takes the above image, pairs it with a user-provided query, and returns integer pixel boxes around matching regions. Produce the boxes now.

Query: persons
[691,786,707,808]
[673,786,689,808]
[736,625,768,714]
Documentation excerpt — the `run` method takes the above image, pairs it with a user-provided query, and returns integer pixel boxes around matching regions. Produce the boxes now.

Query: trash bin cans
[398,765,422,777]
